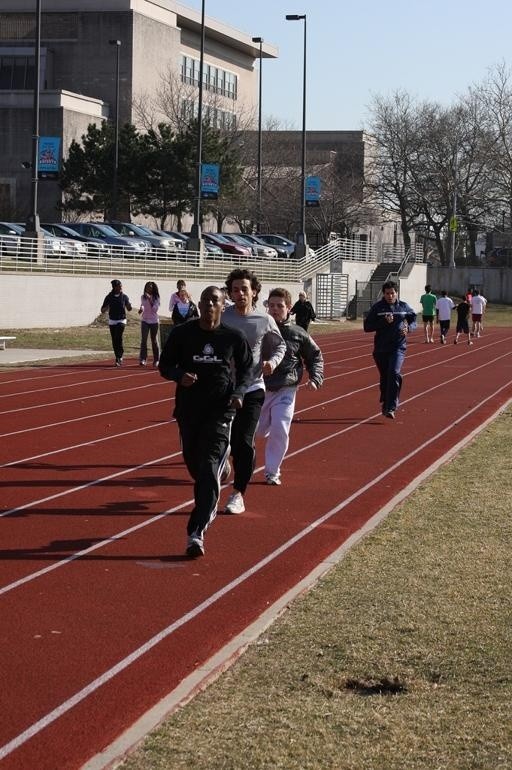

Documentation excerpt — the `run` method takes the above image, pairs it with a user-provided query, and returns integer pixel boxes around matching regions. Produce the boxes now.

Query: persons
[420,284,488,346]
[220,287,233,312]
[158,286,255,557]
[139,282,160,368]
[363,281,417,420]
[169,280,186,312]
[220,268,287,514]
[101,279,132,367]
[172,290,199,327]
[290,290,316,332]
[255,288,324,485]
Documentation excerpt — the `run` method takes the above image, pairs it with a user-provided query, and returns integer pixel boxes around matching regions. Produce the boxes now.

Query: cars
[15,223,88,260]
[137,225,316,264]
[0,222,65,260]
[39,223,110,258]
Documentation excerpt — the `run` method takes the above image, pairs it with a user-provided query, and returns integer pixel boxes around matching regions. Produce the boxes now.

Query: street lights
[107,39,123,220]
[500,210,506,246]
[285,14,309,263]
[252,37,265,235]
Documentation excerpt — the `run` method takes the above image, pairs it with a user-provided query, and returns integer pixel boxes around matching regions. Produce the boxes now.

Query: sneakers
[224,495,246,514]
[186,539,204,557]
[266,474,281,485]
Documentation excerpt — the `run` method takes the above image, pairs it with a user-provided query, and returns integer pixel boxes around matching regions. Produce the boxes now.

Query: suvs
[64,223,152,261]
[485,245,511,268]
[109,222,185,261]
